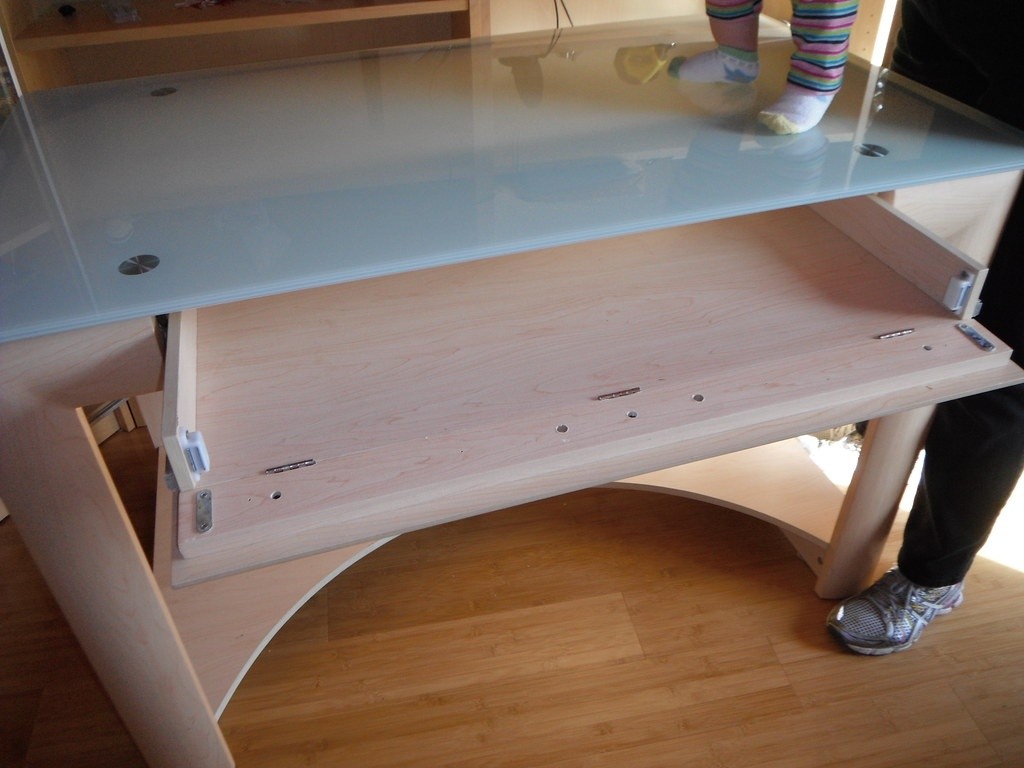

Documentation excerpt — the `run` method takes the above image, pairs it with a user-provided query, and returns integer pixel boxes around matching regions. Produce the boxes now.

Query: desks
[0,52,96,351]
[24,0,1024,336]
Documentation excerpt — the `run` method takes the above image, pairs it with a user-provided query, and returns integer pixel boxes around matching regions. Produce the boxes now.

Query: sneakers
[825,563,964,655]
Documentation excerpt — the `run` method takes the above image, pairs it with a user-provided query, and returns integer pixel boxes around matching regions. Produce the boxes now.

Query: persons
[829,1,1023,675]
[666,0,858,134]
